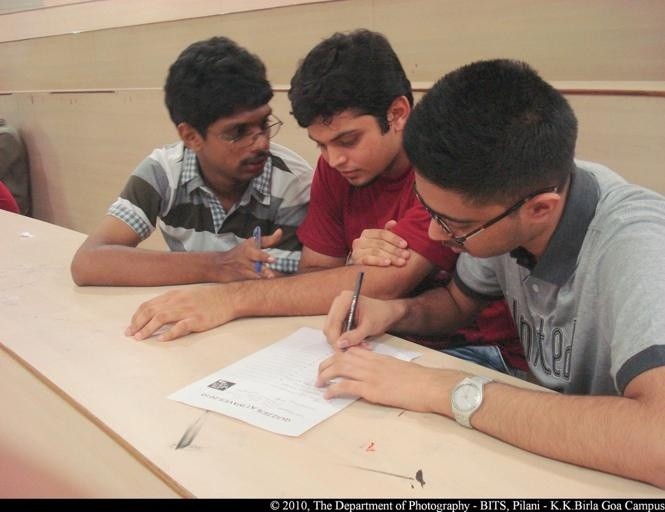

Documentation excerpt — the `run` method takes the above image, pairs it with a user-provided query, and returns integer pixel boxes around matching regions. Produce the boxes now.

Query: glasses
[411,182,560,245]
[203,110,283,151]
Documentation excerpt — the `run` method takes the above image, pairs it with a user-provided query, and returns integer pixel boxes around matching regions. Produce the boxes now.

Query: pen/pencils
[343,272,364,351]
[253,226,261,273]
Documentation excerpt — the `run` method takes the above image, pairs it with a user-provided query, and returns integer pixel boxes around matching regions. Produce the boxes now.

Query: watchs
[450,372,497,431]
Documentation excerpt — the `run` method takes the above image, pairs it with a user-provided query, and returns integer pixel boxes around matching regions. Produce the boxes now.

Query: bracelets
[344,250,354,265]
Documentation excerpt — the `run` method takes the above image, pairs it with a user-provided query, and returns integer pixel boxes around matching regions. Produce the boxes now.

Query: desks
[0,209,665,498]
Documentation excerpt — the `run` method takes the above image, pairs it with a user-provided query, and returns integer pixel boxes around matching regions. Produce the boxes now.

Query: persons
[70,35,316,289]
[122,23,550,390]
[0,118,31,217]
[313,57,665,494]
